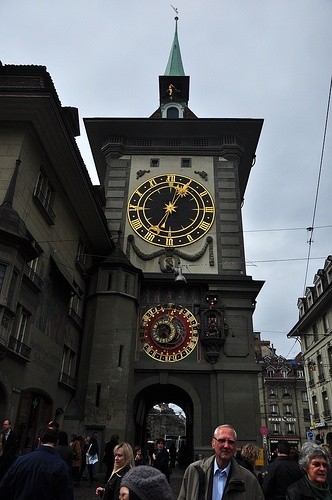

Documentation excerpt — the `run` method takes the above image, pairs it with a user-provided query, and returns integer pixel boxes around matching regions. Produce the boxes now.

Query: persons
[0,430,74,500]
[177,424,264,500]
[0,423,332,488]
[119,465,173,500]
[96,443,135,500]
[286,443,332,500]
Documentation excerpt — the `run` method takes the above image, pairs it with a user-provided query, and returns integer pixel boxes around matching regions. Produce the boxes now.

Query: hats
[120,465,172,500]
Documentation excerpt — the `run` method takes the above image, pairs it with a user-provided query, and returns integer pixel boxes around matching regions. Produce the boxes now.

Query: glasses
[212,437,238,444]
[309,461,329,468]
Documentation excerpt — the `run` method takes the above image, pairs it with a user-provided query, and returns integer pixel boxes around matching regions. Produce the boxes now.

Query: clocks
[139,303,200,363]
[128,173,216,248]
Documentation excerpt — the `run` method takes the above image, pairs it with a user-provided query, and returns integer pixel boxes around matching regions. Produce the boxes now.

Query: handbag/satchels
[86,444,98,464]
